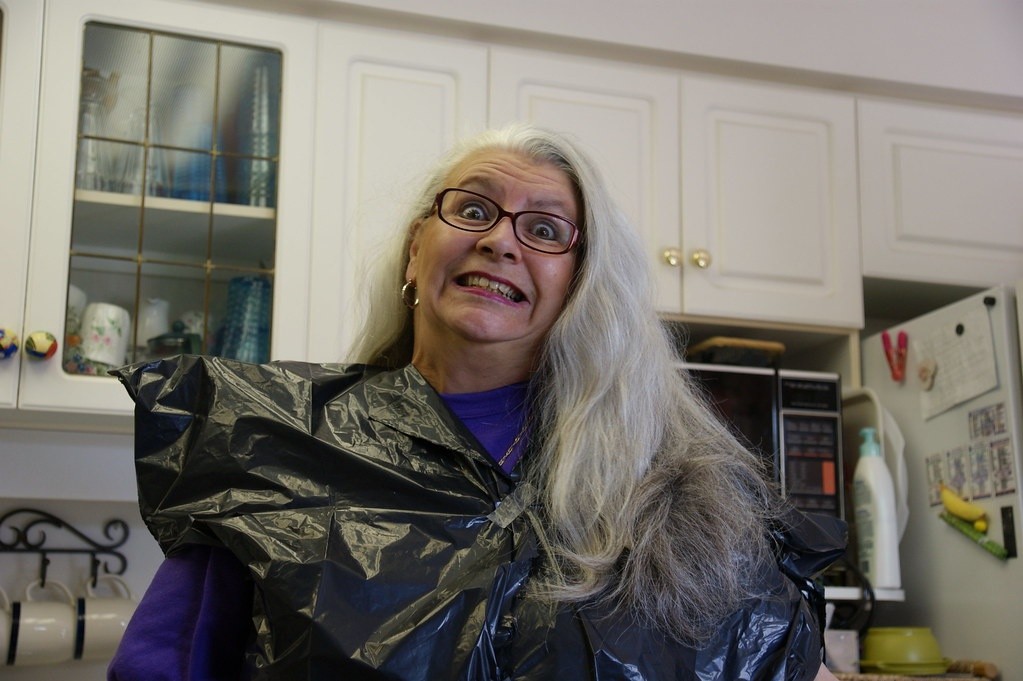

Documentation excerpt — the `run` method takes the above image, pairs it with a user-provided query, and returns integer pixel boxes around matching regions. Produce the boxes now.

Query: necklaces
[499,411,535,466]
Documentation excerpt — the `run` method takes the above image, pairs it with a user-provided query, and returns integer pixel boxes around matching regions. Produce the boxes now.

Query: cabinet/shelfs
[0,0,1022,426]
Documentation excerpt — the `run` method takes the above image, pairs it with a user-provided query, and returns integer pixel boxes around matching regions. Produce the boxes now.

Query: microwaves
[677,364,846,524]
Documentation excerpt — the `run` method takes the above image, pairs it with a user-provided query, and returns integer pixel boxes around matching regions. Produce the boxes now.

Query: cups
[824,630,859,674]
[68,275,271,368]
[0,574,140,667]
[75,65,277,208]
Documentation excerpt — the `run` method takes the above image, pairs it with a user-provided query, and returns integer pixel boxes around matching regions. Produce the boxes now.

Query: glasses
[427,188,586,255]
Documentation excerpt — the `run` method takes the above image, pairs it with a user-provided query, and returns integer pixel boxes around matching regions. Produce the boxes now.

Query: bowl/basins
[862,626,944,660]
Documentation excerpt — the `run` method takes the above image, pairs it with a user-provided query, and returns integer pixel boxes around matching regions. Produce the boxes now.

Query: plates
[860,657,957,675]
[685,335,786,369]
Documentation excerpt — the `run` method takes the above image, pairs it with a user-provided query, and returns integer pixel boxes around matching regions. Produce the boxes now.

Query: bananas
[940,479,986,520]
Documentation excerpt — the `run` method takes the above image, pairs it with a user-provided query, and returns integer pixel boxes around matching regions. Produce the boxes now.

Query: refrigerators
[860,285,1023,681]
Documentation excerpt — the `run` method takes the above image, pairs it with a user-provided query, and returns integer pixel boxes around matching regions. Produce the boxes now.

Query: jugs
[130,297,171,349]
[146,319,207,362]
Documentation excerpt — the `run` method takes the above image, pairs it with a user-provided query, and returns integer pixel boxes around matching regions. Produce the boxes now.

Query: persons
[106,126,823,680]
